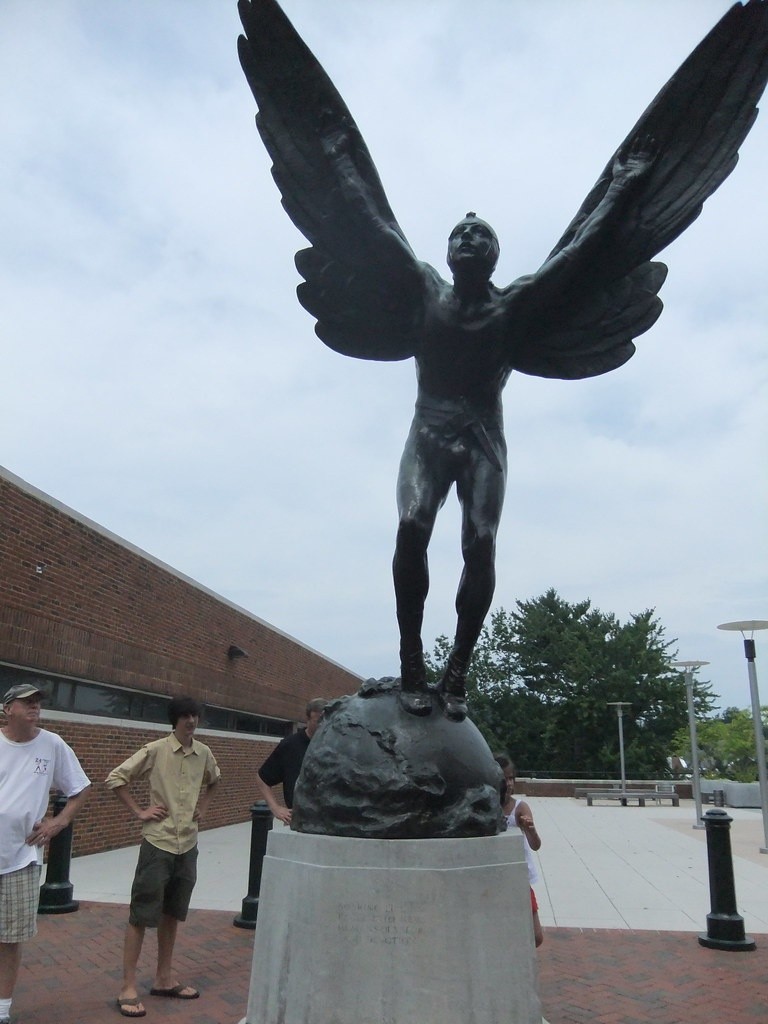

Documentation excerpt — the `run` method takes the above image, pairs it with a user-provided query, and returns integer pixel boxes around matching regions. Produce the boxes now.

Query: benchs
[575,788,680,807]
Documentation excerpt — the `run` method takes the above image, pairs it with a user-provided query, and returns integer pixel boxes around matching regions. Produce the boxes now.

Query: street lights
[666,661,711,829]
[717,620,768,854]
[607,702,634,806]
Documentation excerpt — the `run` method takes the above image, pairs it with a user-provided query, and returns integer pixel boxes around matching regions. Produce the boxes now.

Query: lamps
[228,645,250,660]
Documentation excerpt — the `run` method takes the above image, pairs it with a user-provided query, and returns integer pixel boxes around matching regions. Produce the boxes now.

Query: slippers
[117,998,146,1016]
[150,981,200,999]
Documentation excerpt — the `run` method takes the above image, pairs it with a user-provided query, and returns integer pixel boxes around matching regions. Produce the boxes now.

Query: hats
[306,698,329,712]
[3,683,47,704]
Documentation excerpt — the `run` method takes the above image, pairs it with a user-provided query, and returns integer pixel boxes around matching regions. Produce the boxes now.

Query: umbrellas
[666,756,688,769]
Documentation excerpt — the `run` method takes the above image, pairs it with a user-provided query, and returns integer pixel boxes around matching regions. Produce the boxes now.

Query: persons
[317,106,665,722]
[489,752,550,1024]
[256,697,329,828]
[0,684,92,1024]
[105,695,222,1018]
